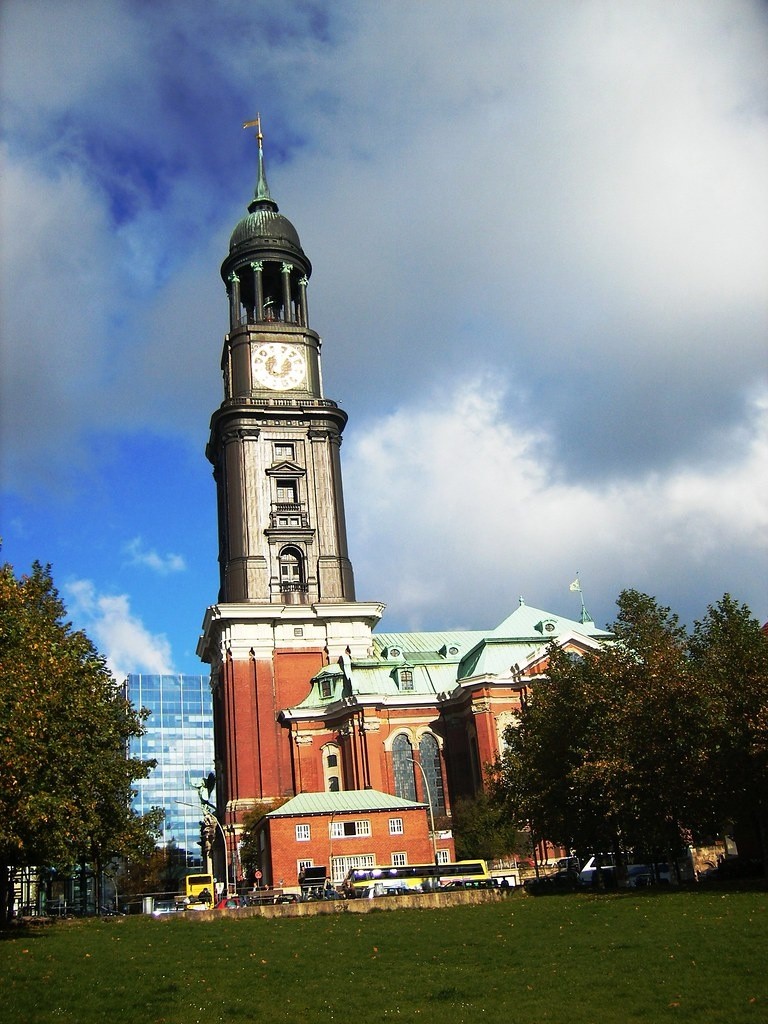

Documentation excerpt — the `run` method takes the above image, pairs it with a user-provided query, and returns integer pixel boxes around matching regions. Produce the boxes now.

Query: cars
[275,894,304,904]
[210,898,243,909]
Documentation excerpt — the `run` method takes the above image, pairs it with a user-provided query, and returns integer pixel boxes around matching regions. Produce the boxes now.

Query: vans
[441,879,499,890]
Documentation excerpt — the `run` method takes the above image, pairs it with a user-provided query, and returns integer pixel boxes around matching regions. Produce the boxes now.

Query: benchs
[247,890,283,905]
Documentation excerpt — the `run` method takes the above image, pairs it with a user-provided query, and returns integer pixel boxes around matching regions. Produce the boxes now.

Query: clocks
[252,343,306,391]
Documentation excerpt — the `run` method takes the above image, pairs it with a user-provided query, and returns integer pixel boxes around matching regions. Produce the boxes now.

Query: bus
[343,859,492,889]
[185,874,215,910]
[579,848,696,888]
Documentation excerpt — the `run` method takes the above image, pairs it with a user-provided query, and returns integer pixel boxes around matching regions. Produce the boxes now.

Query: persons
[186,867,359,906]
[500,876,509,887]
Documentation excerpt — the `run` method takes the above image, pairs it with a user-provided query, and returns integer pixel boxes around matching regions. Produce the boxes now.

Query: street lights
[175,800,229,900]
[406,757,441,892]
[330,810,337,890]
